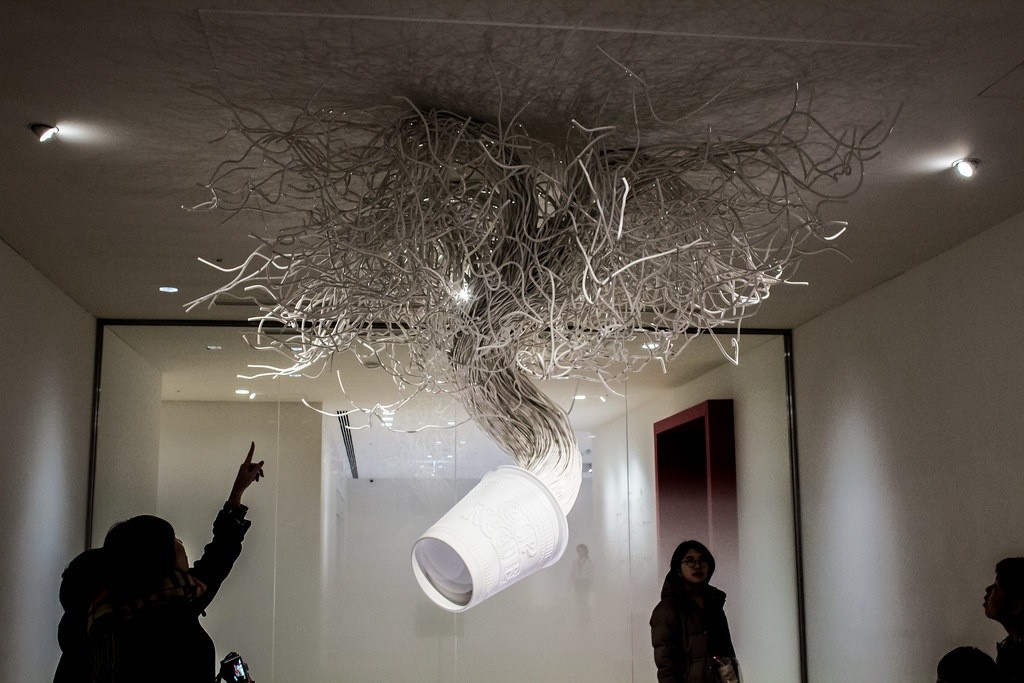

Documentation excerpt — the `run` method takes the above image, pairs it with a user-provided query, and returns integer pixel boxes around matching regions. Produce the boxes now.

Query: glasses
[679,557,710,569]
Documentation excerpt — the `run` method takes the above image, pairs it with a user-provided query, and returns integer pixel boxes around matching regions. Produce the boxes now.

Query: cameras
[220,654,248,683]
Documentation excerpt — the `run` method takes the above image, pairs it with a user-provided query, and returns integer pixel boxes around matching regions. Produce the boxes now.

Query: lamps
[952,158,980,177]
[29,123,56,143]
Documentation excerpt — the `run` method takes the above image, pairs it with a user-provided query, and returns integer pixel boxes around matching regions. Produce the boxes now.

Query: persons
[983,557,1024,683]
[572,544,592,589]
[650,540,739,683]
[52,440,264,683]
[936,646,996,683]
